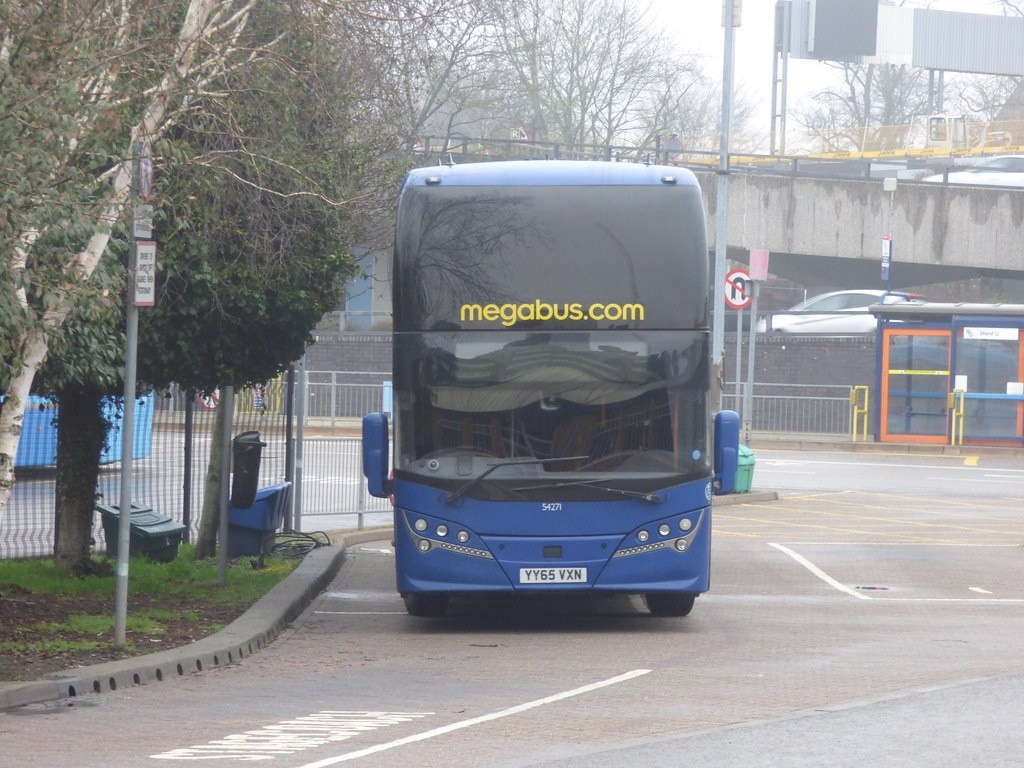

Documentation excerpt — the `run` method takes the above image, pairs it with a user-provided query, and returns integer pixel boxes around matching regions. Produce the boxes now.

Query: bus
[357,158,715,626]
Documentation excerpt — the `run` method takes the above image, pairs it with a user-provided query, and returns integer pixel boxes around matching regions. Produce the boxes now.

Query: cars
[758,290,929,338]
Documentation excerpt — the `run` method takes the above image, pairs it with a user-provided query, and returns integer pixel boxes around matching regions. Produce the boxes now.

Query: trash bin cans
[733,443,754,493]
[95,501,189,561]
[217,481,293,557]
